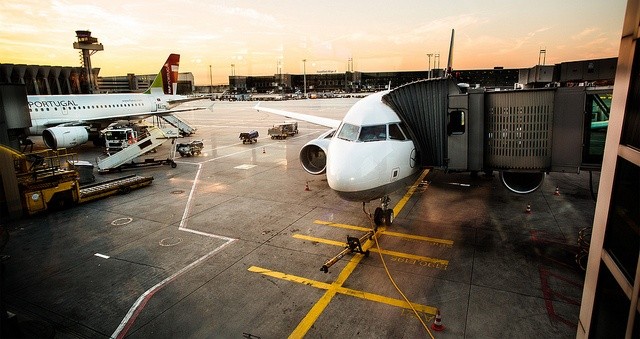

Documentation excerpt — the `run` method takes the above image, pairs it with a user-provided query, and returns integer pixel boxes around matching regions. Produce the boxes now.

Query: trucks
[101,121,157,157]
[267,121,299,140]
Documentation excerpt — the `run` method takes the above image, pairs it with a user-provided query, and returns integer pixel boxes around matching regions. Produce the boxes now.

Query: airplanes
[0,52,216,172]
[252,69,547,226]
